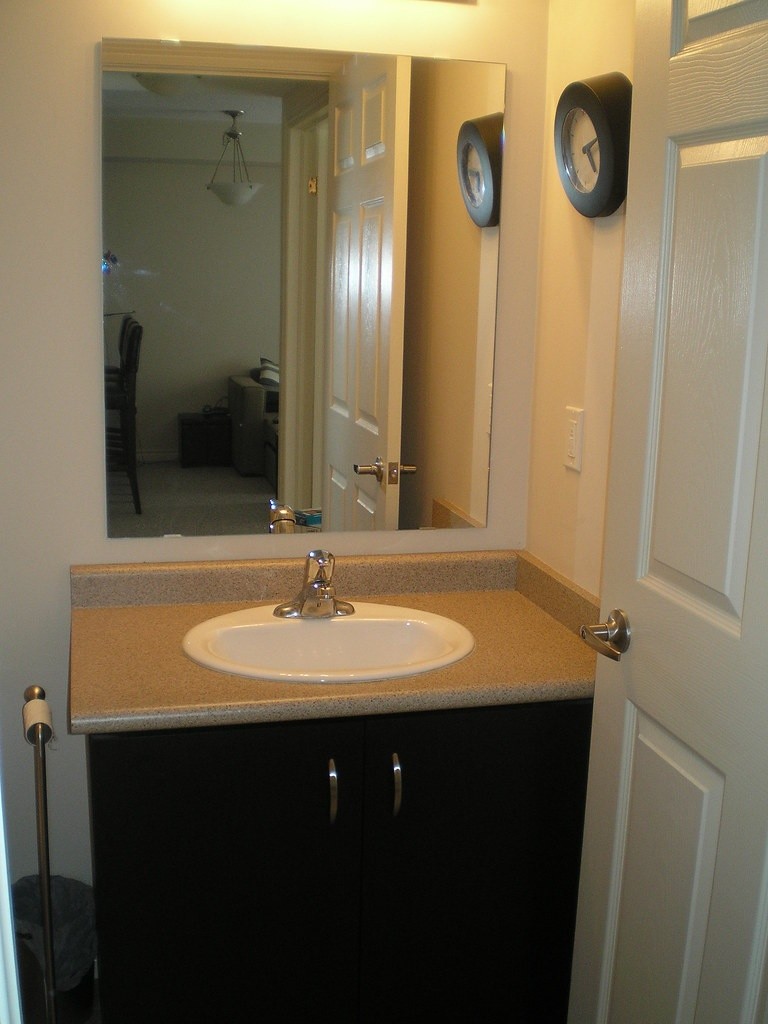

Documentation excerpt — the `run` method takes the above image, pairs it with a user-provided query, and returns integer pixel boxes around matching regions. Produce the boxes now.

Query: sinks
[181,599,476,685]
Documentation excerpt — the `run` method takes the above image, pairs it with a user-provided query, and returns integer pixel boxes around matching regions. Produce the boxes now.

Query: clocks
[457,112,503,227]
[554,71,633,218]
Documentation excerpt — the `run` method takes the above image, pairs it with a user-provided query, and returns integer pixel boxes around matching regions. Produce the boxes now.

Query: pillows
[259,357,279,386]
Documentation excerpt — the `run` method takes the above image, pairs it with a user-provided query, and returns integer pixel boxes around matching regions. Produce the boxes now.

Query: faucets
[298,549,337,619]
[268,496,297,535]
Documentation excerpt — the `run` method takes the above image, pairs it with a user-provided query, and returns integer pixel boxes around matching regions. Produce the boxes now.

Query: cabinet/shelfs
[83,700,597,1024]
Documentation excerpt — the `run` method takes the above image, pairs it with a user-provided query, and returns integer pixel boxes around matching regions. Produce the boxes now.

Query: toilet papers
[21,698,54,747]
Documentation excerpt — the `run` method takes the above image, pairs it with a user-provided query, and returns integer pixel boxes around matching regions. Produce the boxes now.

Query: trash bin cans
[12,873,99,1023]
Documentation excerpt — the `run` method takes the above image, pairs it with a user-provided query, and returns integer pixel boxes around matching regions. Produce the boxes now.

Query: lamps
[205,110,264,206]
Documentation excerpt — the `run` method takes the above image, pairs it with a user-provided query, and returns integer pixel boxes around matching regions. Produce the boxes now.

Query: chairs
[230,374,279,477]
[105,313,143,515]
